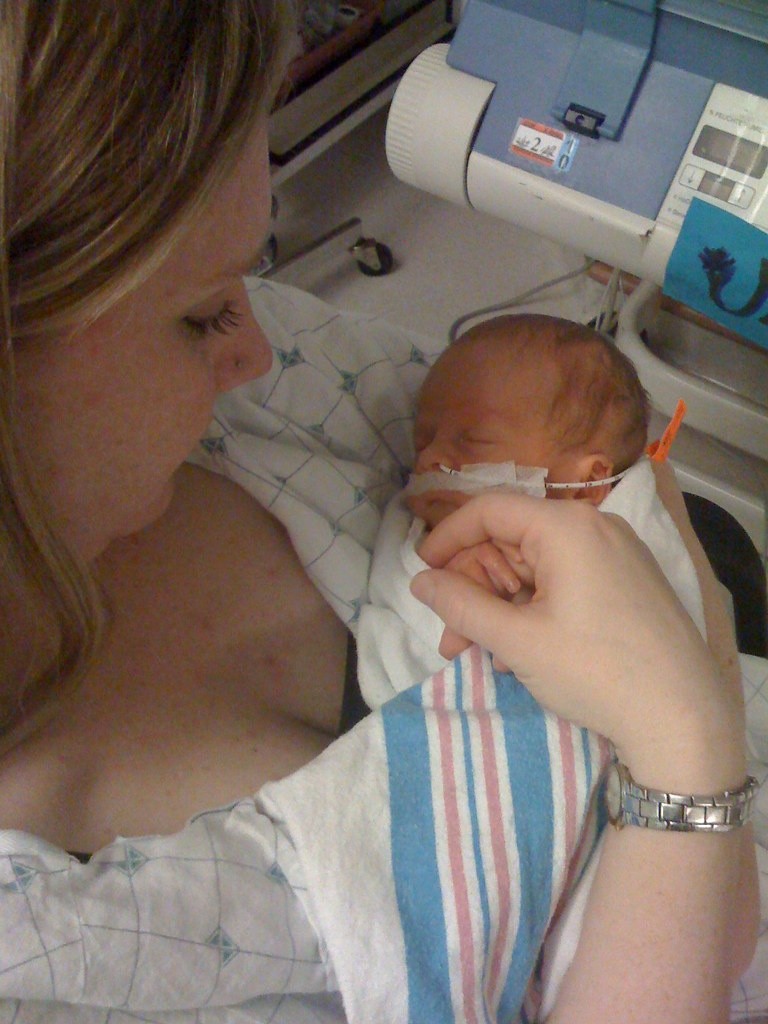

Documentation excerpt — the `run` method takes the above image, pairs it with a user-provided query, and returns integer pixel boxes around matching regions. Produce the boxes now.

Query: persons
[359,315,706,1024]
[0,0,768,1024]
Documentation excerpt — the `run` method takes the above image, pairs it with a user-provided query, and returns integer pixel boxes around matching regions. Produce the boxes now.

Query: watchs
[603,764,759,834]
